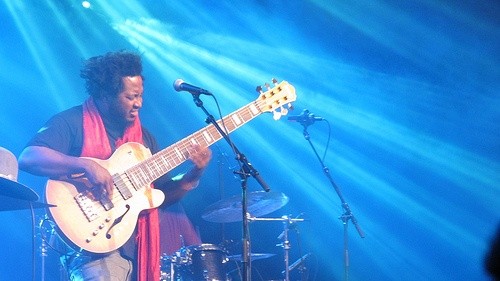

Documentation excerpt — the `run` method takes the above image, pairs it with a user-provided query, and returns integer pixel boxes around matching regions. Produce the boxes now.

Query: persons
[18,50,213,281]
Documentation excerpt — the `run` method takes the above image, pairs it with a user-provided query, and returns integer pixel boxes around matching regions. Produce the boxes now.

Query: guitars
[43,78,297,257]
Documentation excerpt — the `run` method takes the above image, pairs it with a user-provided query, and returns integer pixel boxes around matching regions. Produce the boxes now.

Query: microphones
[173,79,212,95]
[278,213,303,240]
[287,115,324,121]
[282,254,309,275]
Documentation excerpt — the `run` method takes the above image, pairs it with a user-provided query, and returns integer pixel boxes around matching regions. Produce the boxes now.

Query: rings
[203,153,206,159]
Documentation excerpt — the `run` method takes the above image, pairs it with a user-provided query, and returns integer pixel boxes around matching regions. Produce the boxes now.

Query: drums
[170,243,230,281]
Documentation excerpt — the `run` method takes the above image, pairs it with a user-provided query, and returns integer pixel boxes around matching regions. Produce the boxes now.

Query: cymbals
[201,190,289,223]
[227,254,276,261]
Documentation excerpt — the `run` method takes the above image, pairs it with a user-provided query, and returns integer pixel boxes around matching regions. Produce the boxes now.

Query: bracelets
[188,172,203,180]
